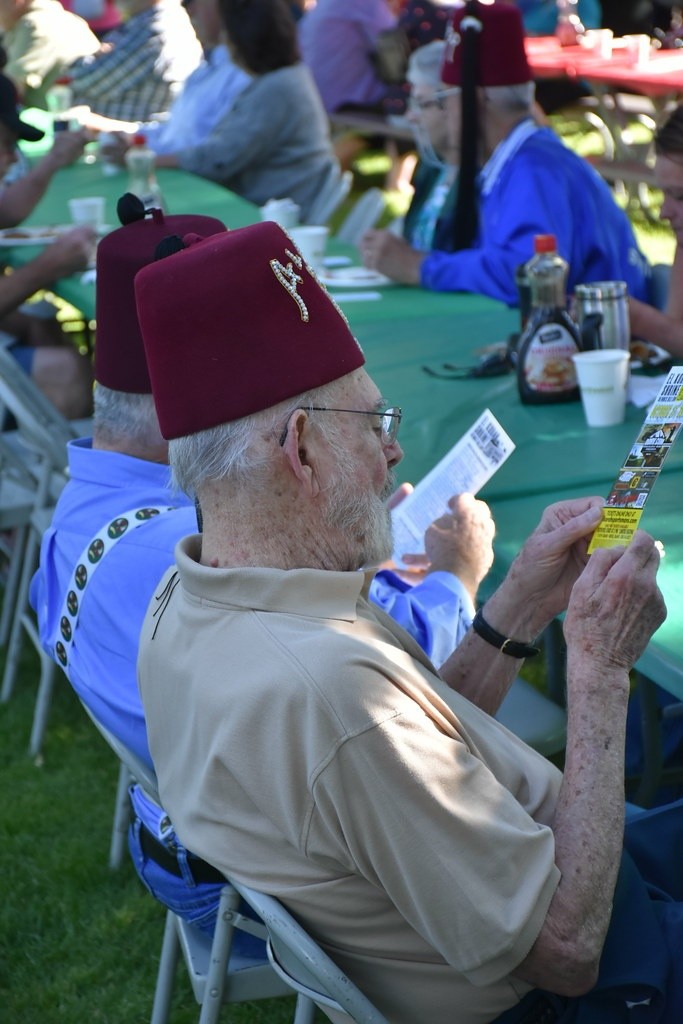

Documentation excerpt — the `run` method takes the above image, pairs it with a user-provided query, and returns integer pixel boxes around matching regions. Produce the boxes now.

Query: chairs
[0,435,66,651]
[300,156,340,227]
[200,871,388,1024]
[338,186,388,246]
[0,344,81,759]
[80,700,316,1024]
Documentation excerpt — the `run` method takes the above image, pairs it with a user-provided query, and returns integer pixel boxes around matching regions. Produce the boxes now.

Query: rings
[365,250,371,257]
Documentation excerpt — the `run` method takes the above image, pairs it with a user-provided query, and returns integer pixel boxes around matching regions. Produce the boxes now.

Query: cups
[259,206,302,227]
[67,196,105,233]
[570,348,631,428]
[585,29,613,59]
[46,91,72,113]
[287,225,330,277]
[623,33,651,70]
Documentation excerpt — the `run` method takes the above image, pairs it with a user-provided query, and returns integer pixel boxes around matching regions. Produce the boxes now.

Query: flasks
[574,280,631,352]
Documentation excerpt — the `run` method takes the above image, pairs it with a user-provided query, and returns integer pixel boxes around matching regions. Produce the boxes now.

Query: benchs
[329,110,655,186]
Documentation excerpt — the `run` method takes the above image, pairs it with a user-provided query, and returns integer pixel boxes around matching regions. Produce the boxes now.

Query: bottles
[97,130,123,177]
[514,234,586,405]
[124,131,168,218]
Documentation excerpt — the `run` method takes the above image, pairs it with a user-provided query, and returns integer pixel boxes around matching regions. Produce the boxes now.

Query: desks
[0,101,683,501]
[474,468,683,807]
[523,33,683,231]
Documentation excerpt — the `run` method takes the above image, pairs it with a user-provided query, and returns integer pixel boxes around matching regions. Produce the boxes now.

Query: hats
[439,1,531,84]
[132,222,364,439]
[95,208,228,395]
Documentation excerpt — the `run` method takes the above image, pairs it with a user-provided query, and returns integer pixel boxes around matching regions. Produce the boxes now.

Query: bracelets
[471,608,544,660]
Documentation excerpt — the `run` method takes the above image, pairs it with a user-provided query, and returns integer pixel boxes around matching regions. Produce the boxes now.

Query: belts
[129,796,224,884]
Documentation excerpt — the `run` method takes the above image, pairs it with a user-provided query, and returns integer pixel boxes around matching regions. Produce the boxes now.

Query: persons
[135,221,683,1024]
[30,196,644,969]
[0,0,330,422]
[357,0,654,311]
[565,101,683,360]
[292,0,683,196]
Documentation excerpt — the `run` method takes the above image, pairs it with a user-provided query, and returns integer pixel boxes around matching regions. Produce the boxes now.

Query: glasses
[408,99,439,114]
[435,87,461,107]
[278,405,402,446]
